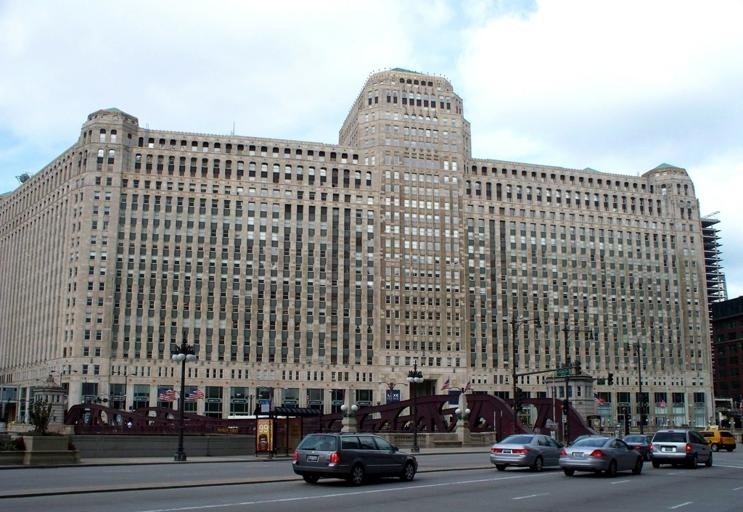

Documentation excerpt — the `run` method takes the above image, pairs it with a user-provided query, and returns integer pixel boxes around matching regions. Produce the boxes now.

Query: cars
[624,435,654,461]
[698,430,737,452]
[292,430,419,488]
[559,434,644,478]
[648,428,713,469]
[489,433,563,472]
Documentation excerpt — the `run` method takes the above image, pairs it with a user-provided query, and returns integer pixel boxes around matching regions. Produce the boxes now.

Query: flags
[189,389,204,400]
[441,379,449,390]
[465,382,471,391]
[159,387,177,401]
[593,393,604,405]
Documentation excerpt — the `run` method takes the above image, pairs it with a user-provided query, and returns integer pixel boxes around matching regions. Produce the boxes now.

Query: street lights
[170,342,197,461]
[406,359,424,452]
